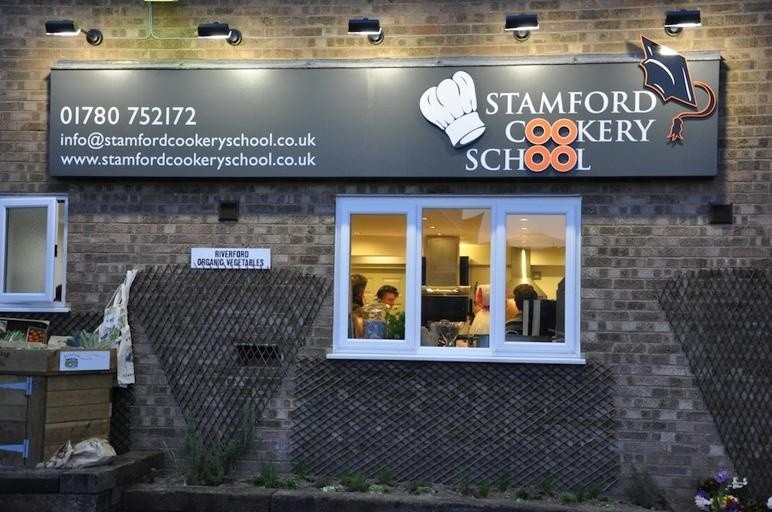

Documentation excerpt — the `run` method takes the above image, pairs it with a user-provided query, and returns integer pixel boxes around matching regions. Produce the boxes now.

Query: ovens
[422,286,471,324]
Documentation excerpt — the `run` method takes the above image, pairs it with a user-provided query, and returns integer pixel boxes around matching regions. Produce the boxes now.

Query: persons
[506,284,539,336]
[374,285,399,310]
[350,274,369,338]
[467,284,490,334]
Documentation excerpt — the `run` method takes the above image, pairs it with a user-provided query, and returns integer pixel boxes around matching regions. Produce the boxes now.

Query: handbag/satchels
[46,436,118,470]
[91,269,144,390]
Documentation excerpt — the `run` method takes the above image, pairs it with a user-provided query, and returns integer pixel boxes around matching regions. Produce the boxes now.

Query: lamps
[503,13,539,40]
[44,20,101,48]
[663,10,702,38]
[196,22,242,45]
[347,18,384,46]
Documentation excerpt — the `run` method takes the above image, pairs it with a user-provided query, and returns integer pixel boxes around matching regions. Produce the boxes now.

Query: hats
[637,34,700,110]
[418,69,487,150]
